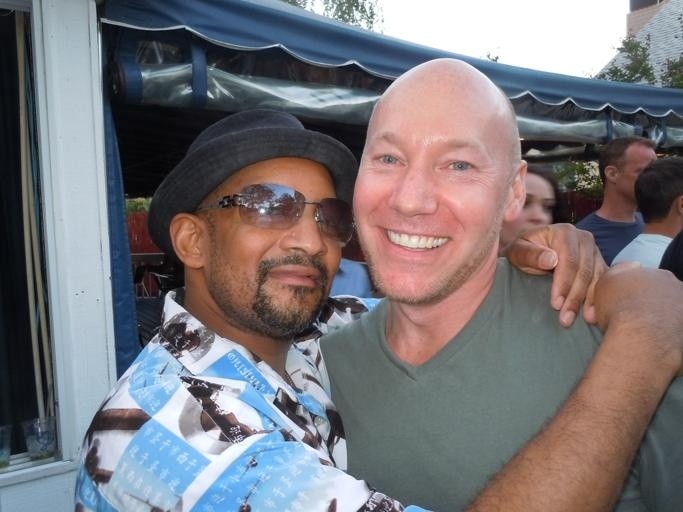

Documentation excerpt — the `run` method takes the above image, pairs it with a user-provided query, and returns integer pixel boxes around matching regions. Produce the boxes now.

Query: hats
[146,108,360,256]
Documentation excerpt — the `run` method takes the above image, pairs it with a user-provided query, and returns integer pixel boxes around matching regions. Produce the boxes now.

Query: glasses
[192,182,354,241]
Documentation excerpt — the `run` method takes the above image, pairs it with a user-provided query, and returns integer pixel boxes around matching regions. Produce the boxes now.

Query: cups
[18,414,56,460]
[0,424,13,469]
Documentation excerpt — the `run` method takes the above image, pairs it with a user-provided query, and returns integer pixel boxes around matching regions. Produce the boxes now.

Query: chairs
[132,255,178,300]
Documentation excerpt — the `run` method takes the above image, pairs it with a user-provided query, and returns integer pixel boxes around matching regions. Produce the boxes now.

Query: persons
[327,254,378,298]
[73,105,681,510]
[659,218,681,287]
[572,133,653,270]
[319,58,680,510]
[607,154,682,269]
[500,162,564,265]
